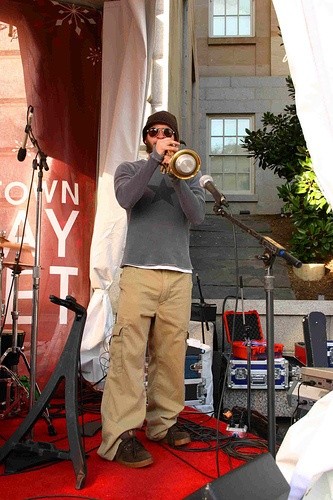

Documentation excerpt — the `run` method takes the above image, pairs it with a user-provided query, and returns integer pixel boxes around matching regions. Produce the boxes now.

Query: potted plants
[238,74,333,282]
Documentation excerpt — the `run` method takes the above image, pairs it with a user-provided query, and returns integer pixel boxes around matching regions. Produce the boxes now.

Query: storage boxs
[223,353,289,390]
[184,345,208,406]
[223,309,283,360]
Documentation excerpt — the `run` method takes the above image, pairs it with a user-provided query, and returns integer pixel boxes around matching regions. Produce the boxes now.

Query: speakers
[183,452,293,500]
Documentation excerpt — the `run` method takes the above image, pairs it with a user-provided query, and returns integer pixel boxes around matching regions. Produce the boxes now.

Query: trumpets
[164,143,200,181]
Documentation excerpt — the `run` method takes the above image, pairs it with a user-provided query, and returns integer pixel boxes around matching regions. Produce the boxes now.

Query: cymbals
[0,238,34,252]
[2,261,43,273]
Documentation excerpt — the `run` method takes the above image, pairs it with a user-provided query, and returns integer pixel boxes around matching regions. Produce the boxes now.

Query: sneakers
[114,435,153,468]
[166,425,191,448]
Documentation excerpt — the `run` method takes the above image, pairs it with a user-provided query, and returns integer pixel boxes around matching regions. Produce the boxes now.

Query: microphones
[199,175,230,208]
[18,106,33,161]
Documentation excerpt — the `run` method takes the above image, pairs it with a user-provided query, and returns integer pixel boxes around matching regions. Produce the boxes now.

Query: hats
[143,111,178,142]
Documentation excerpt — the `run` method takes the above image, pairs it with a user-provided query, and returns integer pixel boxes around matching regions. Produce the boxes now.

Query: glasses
[145,128,175,138]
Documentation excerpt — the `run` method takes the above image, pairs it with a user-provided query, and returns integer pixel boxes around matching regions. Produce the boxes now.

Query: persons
[95,110,207,468]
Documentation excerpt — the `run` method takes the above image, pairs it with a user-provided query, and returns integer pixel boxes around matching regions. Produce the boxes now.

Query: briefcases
[225,311,283,359]
[227,357,289,391]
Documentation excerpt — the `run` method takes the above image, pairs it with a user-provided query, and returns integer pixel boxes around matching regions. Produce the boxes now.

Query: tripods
[0,125,57,435]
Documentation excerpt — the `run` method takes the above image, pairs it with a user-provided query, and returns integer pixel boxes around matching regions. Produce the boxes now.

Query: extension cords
[227,424,248,433]
